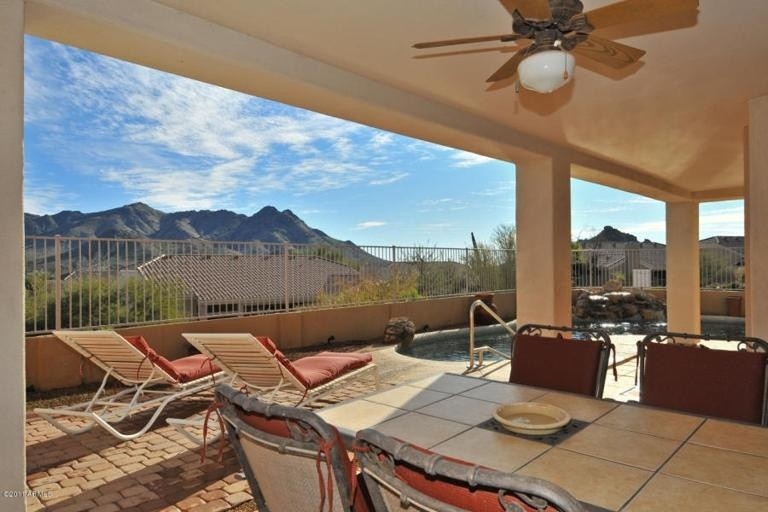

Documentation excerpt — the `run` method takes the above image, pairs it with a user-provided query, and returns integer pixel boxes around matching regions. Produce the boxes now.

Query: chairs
[212,385,372,511]
[32,327,229,441]
[635,331,768,429]
[164,333,377,446]
[508,325,618,398]
[355,429,587,512]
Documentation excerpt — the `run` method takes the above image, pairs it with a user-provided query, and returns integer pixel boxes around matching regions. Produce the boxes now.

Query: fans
[411,0,699,94]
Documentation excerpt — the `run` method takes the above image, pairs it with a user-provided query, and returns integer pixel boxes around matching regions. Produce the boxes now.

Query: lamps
[513,0,596,95]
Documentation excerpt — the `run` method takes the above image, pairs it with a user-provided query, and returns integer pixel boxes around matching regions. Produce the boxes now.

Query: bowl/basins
[492,402,571,436]
